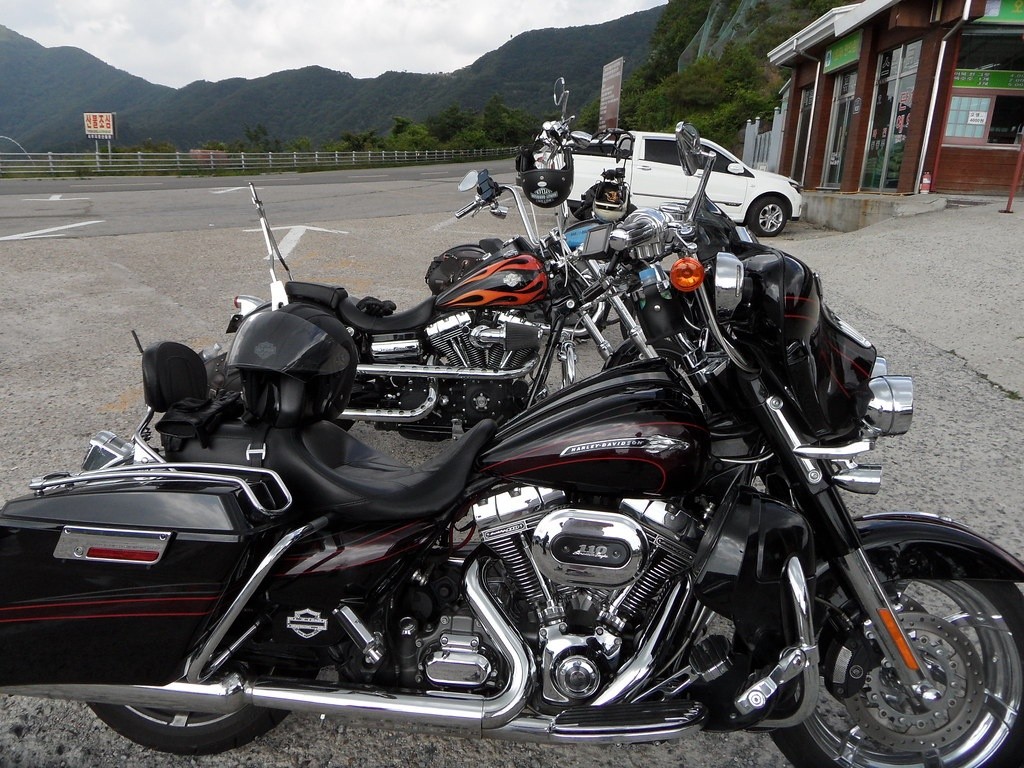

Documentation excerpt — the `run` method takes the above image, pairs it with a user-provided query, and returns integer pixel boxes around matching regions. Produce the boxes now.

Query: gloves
[357,297,397,317]
[155,387,244,448]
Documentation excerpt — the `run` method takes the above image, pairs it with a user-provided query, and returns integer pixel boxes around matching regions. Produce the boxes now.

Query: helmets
[224,302,359,428]
[593,183,629,223]
[515,148,574,209]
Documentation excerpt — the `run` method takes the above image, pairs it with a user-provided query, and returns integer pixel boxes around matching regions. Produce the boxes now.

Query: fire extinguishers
[919,171,932,194]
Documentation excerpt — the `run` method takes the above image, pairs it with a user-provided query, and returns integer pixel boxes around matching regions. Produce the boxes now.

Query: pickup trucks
[530,130,803,239]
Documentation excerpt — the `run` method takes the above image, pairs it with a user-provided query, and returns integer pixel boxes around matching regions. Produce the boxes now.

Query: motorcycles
[0,120,1023,768]
